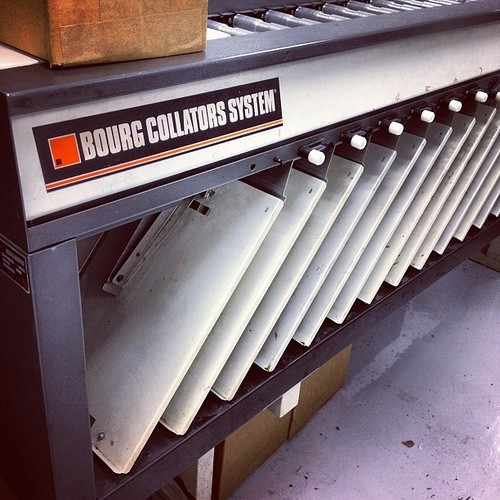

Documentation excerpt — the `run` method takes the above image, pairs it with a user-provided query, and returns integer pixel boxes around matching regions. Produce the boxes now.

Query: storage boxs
[0,0,207,65]
[210,396,291,499]
[289,343,353,441]
